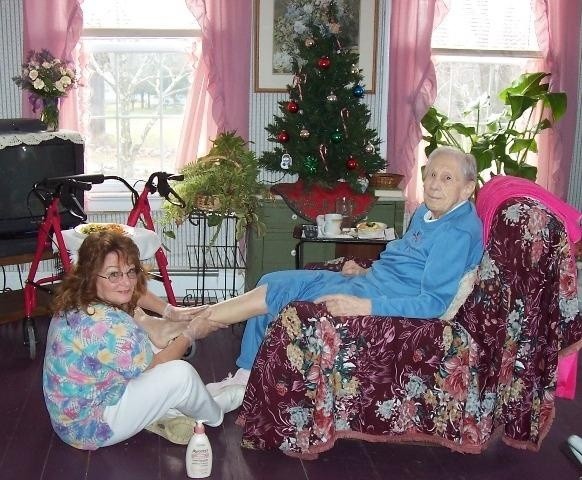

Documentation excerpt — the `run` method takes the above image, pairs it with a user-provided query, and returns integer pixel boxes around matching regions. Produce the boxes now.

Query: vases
[39,95,60,132]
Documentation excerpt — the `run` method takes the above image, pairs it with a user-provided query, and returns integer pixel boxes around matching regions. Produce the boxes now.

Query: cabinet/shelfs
[243,183,408,294]
[0,251,61,325]
[182,209,246,331]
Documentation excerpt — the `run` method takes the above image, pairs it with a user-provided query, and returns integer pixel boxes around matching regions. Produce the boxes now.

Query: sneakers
[144,416,194,446]
[206,376,247,414]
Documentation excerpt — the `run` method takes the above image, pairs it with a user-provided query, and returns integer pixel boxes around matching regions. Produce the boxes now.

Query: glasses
[98,268,138,282]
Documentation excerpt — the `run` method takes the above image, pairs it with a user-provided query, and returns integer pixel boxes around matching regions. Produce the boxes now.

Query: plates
[74,223,134,236]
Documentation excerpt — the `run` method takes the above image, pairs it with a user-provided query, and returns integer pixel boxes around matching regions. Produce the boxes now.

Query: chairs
[236,176,566,461]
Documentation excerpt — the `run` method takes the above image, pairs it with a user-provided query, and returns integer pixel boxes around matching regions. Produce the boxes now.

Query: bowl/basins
[356,222,388,235]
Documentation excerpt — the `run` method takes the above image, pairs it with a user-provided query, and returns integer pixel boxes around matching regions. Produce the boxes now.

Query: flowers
[11,47,78,132]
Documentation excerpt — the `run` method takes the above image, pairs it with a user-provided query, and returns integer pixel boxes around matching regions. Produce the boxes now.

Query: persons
[134,147,486,395]
[43,231,246,451]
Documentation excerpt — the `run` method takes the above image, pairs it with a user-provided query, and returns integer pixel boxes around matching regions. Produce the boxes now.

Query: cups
[322,214,343,235]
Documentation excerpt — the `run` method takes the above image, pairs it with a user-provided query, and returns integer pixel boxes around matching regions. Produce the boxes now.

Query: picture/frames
[253,0,379,94]
